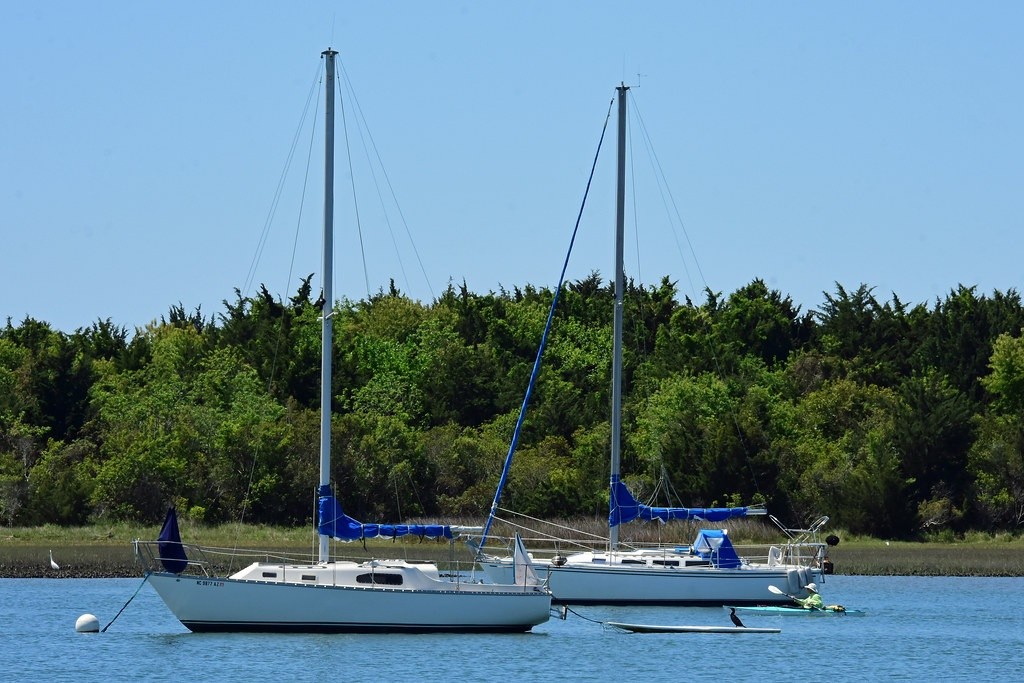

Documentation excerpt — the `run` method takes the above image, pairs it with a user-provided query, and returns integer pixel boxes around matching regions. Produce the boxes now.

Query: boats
[723,603,867,617]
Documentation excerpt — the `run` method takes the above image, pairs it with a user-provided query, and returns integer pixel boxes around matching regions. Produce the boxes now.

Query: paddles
[768,585,821,611]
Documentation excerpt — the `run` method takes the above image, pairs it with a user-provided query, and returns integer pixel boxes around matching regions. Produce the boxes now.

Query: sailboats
[127,45,557,633]
[476,84,834,609]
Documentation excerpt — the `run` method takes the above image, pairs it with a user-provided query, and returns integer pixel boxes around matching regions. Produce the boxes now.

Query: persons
[790,582,823,610]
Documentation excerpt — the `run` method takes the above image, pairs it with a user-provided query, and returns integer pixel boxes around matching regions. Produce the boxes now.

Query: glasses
[807,588,809,590]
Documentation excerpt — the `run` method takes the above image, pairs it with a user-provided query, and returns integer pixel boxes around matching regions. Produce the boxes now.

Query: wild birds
[50,549,59,570]
[730,607,747,628]
[885,542,889,546]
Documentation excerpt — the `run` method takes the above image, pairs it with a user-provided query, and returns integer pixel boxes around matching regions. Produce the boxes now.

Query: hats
[804,583,818,594]
